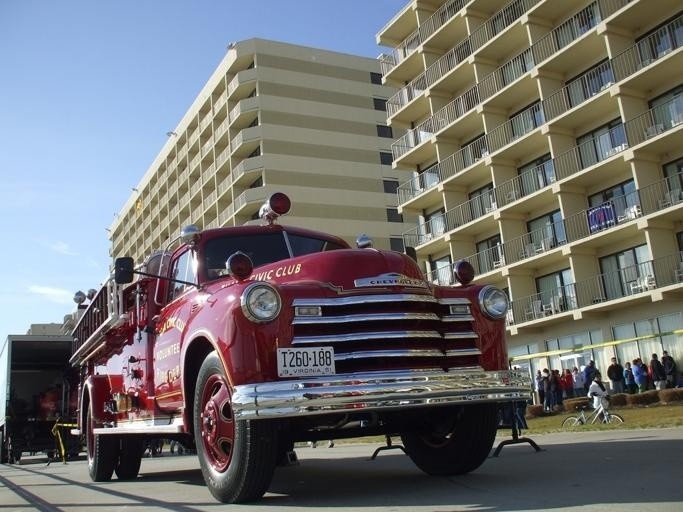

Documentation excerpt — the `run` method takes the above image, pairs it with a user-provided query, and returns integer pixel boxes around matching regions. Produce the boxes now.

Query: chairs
[616,205,638,223]
[503,190,519,204]
[522,295,561,320]
[629,272,657,295]
[521,237,551,258]
[657,189,682,209]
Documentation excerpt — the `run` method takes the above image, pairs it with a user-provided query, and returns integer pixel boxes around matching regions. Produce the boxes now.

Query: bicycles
[560,394,624,427]
[143,438,162,453]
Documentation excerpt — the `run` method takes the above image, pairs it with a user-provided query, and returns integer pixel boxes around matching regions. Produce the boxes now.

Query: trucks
[0,334,80,464]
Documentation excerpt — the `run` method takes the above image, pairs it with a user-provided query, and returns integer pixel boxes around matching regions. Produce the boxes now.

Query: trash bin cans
[525,399,533,404]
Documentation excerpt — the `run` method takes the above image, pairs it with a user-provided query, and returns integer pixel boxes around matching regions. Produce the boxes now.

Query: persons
[311,439,335,448]
[587,370,609,424]
[152,438,192,455]
[607,350,676,395]
[536,360,597,411]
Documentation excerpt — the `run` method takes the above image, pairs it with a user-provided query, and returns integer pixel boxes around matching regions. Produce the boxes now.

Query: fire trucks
[68,192,533,504]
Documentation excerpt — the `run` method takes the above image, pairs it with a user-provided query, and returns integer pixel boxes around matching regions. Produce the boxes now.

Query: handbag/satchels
[539,384,544,390]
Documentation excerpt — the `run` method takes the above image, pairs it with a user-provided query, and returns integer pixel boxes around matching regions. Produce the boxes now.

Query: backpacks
[543,374,550,392]
